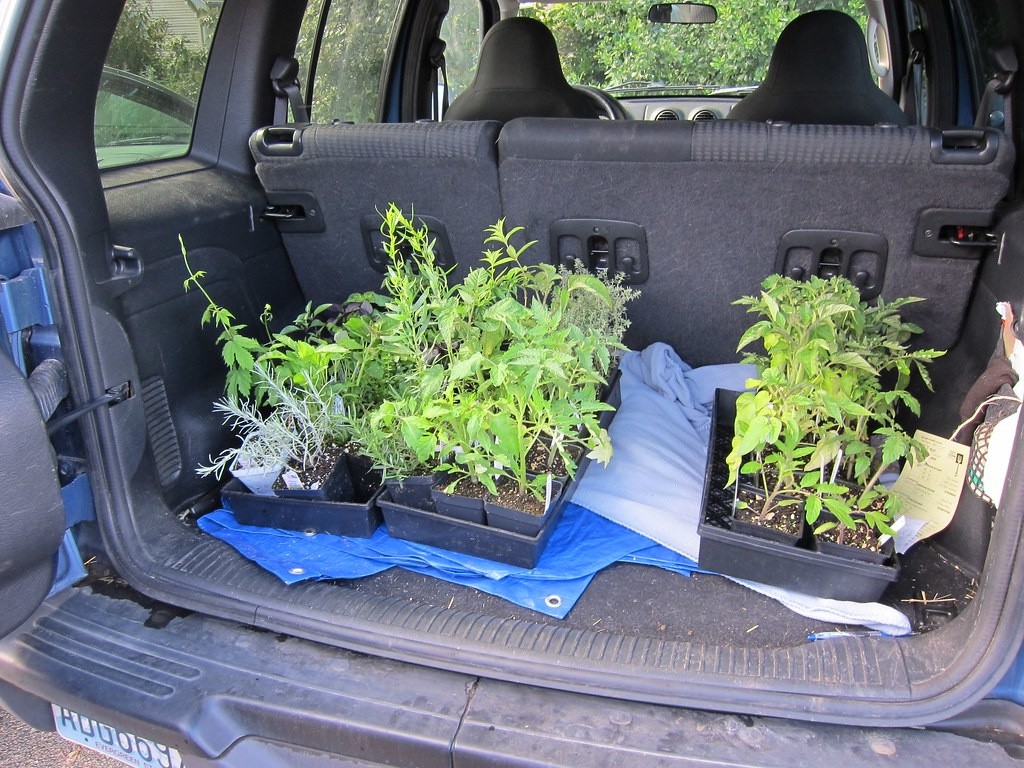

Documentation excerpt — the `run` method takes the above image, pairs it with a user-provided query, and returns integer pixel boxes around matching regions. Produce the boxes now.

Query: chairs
[729,11,907,126]
[444,19,600,120]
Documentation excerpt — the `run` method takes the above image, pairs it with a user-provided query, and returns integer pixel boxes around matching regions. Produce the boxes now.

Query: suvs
[0,0,1024,768]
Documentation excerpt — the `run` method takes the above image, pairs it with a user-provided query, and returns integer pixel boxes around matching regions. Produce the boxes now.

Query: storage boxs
[375,369,625,570]
[219,475,388,538]
[695,387,903,602]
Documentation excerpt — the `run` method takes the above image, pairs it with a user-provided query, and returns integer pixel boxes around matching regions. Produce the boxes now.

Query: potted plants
[178,200,645,539]
[722,270,949,563]
